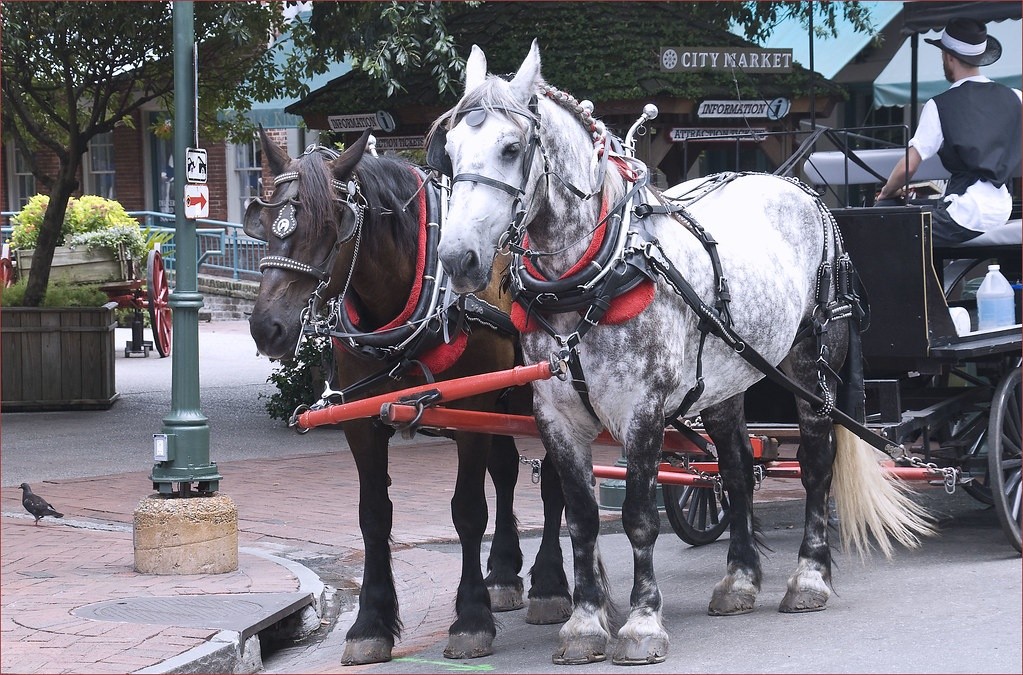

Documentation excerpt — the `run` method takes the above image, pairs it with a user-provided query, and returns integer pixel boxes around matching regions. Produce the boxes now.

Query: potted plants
[0,277,123,411]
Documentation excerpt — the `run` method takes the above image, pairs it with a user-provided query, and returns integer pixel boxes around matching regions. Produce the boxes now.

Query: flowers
[8,192,147,265]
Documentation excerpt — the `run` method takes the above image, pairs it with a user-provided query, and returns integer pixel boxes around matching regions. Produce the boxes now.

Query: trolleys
[0,240,223,358]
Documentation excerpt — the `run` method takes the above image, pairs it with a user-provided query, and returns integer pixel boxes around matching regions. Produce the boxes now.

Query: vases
[18,243,124,285]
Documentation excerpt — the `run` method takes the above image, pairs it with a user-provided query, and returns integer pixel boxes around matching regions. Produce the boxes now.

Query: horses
[247,32,854,665]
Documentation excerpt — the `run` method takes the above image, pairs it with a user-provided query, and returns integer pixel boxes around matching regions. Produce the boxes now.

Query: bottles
[977,265,1015,331]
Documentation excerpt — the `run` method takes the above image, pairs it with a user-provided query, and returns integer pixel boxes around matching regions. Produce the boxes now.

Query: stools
[931,218,1023,309]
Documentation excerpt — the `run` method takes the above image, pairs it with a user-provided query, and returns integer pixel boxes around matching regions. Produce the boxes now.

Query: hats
[924,17,1003,67]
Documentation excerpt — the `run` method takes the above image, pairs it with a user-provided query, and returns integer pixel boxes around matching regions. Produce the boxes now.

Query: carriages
[240,37,1023,669]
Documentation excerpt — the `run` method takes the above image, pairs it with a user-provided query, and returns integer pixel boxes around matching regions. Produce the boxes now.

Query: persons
[874,16,1023,291]
